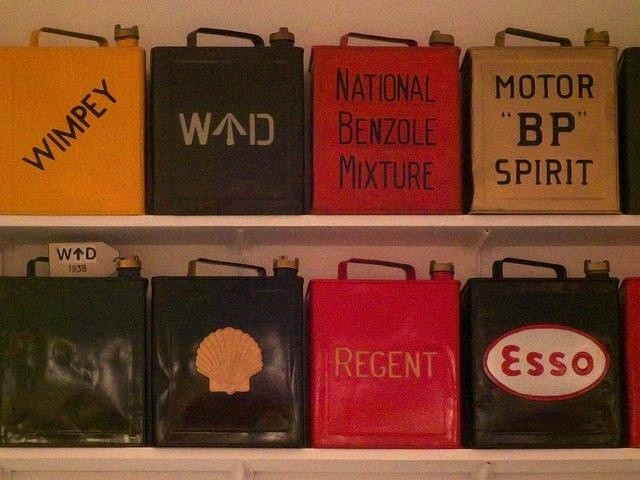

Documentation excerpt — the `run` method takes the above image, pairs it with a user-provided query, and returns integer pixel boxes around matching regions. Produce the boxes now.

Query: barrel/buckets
[309,28,465,215]
[461,257,630,448]
[620,276,640,448]
[146,24,307,215]
[467,27,622,214]
[0,23,148,217]
[0,252,149,448]
[304,258,463,450]
[135,253,310,448]
[618,47,640,215]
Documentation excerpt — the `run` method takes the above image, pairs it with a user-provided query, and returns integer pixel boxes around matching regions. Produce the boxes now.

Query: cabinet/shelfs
[1,215,639,478]
